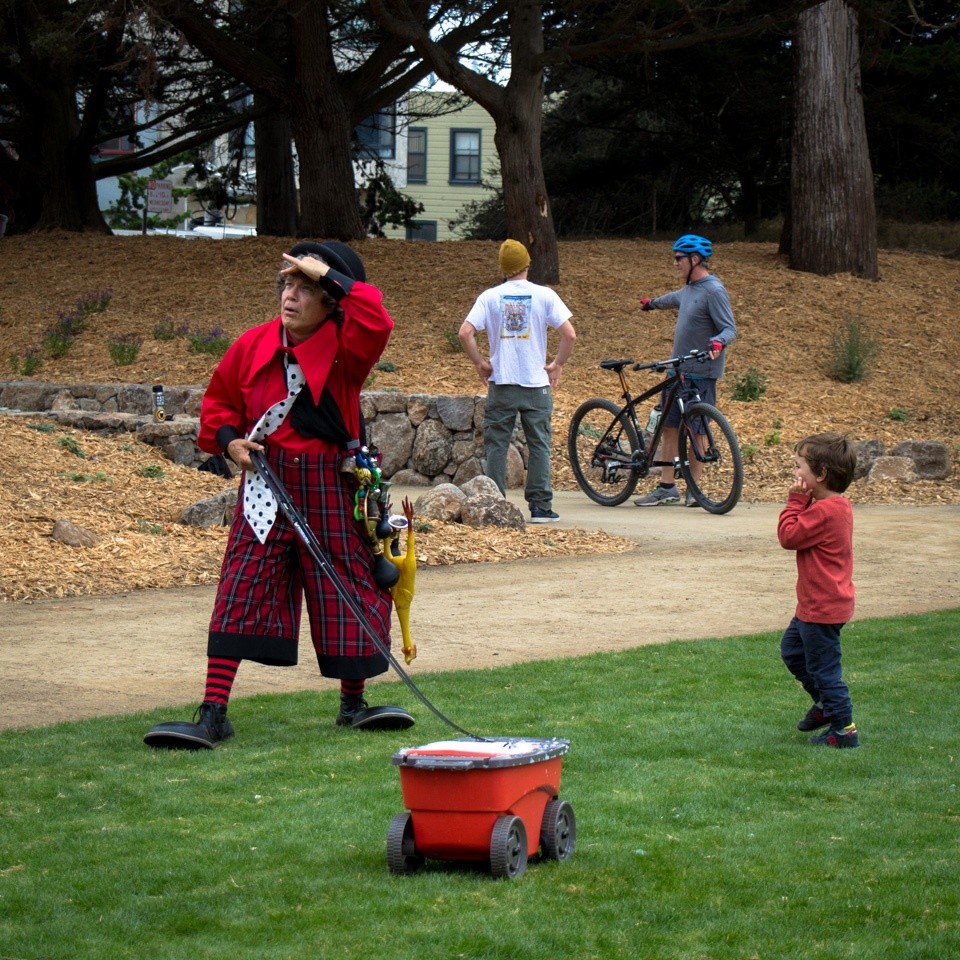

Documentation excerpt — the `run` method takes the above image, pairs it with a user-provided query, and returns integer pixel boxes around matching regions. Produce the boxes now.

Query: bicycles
[567,347,743,514]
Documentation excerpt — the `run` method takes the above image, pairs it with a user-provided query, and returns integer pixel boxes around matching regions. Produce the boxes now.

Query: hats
[500,239,532,278]
[287,240,365,282]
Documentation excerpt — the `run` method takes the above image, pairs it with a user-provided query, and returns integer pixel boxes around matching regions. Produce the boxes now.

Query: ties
[242,363,306,545]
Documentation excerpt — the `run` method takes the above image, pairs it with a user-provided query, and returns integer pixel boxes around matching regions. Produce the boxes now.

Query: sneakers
[529,507,562,523]
[808,726,859,749]
[633,484,680,507]
[798,705,828,731]
[686,487,701,507]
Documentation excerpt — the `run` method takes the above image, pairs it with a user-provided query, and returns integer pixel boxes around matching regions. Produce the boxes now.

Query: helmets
[672,234,713,260]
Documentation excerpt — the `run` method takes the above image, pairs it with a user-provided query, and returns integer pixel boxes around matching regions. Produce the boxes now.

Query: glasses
[675,255,689,261]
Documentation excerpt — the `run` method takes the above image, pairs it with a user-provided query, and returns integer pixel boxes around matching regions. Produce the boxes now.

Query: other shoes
[335,694,416,729]
[142,702,233,748]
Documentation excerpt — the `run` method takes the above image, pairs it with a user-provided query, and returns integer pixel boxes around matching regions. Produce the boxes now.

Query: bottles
[645,404,663,434]
[152,385,165,423]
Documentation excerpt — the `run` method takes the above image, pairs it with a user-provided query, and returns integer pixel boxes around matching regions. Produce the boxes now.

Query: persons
[777,432,861,748]
[458,238,579,524]
[633,234,739,510]
[144,239,414,750]
